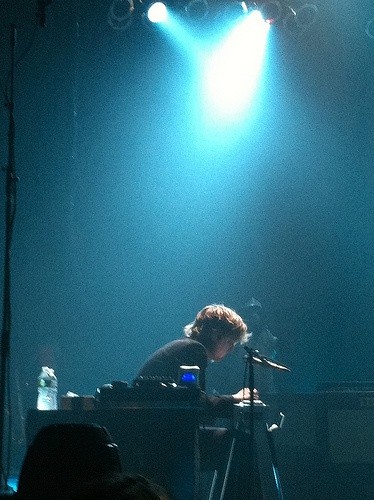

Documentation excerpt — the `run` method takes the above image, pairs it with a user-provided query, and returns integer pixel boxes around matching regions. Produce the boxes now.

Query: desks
[25,403,273,500]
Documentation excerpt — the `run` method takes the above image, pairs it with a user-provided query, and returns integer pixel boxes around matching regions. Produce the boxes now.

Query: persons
[136,303,262,407]
[71,475,168,500]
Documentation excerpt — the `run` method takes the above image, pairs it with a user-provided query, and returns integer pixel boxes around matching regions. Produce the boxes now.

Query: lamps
[146,0,169,24]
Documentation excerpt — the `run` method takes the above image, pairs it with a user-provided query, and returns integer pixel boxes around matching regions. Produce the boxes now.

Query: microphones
[243,355,293,376]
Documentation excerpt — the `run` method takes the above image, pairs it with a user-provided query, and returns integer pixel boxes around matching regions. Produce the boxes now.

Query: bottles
[36,366,53,410]
[49,368,58,410]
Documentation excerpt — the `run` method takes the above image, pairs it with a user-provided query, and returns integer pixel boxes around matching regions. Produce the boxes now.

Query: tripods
[207,359,285,499]
